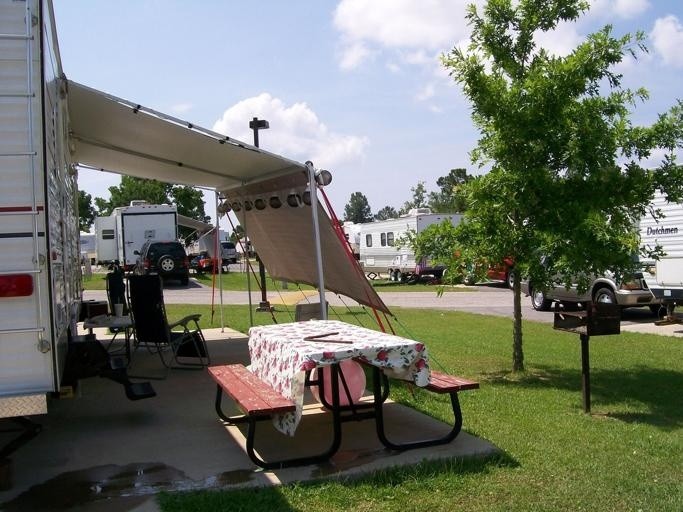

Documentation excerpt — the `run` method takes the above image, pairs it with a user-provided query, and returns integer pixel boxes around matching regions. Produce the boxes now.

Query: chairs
[106,271,211,371]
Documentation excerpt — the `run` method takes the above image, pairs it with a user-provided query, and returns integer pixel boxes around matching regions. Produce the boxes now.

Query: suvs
[132,240,189,286]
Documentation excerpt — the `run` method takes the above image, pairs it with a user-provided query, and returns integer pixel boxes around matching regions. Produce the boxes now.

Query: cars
[217,241,238,265]
[459,238,672,318]
[189,251,211,268]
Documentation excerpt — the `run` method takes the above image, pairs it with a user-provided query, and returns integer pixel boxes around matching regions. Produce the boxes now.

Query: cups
[114,303,124,318]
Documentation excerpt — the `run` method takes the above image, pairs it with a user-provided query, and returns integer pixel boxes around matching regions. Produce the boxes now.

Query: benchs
[209,363,298,470]
[394,368,479,451]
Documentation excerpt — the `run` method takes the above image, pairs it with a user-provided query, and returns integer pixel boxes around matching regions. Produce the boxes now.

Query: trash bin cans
[87,301,108,319]
[78,300,95,321]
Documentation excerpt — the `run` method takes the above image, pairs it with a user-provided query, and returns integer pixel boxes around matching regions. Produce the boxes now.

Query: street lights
[249,117,275,311]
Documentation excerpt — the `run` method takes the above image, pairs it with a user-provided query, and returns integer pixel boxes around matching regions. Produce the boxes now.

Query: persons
[105,259,129,316]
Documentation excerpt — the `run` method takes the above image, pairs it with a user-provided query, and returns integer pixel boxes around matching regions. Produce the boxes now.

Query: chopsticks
[303,332,352,345]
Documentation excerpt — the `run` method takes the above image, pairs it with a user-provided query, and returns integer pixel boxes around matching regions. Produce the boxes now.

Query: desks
[247,319,432,465]
